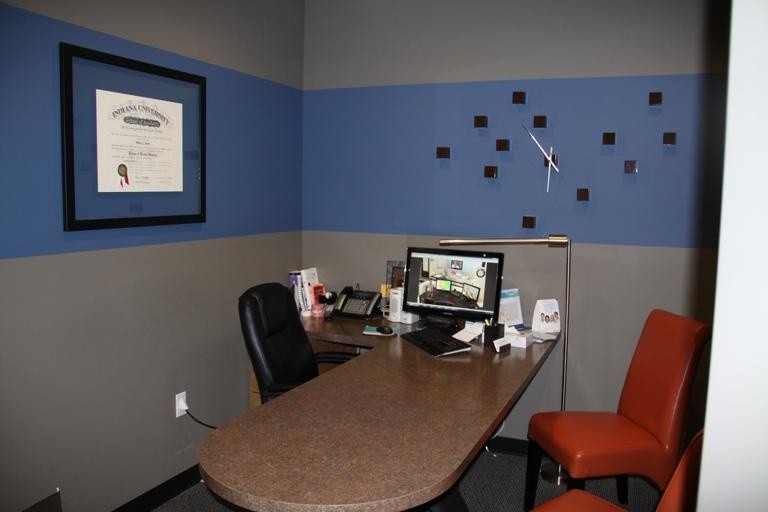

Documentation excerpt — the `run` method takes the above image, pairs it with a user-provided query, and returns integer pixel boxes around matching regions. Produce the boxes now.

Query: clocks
[436,91,676,228]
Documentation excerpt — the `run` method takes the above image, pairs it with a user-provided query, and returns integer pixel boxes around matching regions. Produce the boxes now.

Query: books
[300,266,320,309]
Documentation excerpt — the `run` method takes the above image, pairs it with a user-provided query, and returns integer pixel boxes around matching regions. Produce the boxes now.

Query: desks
[198,311,562,512]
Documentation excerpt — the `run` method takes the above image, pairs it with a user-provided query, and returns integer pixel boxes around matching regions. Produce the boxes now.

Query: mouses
[377,326,393,334]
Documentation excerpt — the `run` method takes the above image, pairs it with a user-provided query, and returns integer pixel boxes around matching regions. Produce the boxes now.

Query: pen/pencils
[485,317,495,327]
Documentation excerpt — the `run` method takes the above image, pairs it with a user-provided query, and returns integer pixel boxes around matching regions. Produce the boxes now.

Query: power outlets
[175,390,188,419]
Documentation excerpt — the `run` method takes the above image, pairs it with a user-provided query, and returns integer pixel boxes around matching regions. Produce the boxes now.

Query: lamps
[439,235,571,486]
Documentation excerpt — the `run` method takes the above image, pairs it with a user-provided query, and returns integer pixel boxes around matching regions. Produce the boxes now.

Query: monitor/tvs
[402,247,504,334]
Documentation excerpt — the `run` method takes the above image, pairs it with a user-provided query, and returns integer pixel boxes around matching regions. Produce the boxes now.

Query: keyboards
[401,327,471,358]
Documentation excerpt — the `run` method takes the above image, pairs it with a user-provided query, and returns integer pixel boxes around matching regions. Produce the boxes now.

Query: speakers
[389,287,420,324]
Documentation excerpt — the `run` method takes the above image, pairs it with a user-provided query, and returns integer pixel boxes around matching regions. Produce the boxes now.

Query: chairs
[522,309,712,512]
[524,427,703,512]
[238,282,319,404]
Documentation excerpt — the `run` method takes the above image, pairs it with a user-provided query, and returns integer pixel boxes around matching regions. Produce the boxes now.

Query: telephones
[334,286,382,318]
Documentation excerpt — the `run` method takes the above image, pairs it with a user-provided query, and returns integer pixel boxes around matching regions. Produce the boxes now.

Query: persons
[540,310,560,323]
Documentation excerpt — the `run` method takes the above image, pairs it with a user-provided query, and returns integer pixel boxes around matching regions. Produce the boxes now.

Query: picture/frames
[59,41,207,232]
[387,261,405,306]
